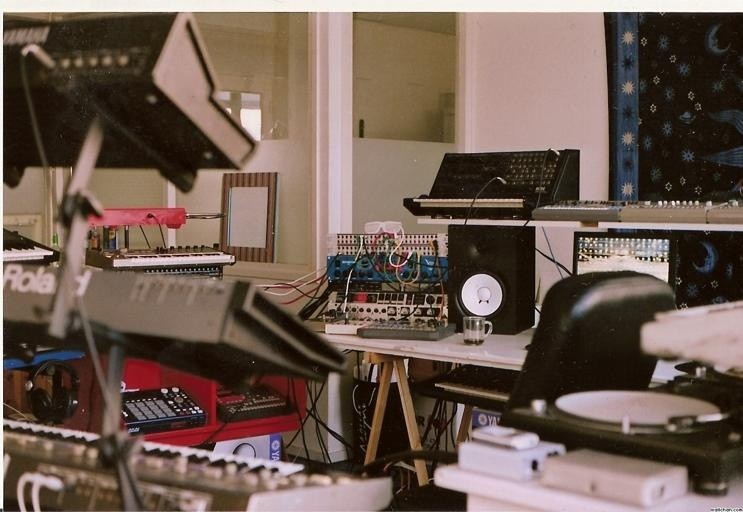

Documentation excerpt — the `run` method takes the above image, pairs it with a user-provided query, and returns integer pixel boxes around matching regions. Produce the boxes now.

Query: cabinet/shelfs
[49,233,64,269]
[83,224,100,252]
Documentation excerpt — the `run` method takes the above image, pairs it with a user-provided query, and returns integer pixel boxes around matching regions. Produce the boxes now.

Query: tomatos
[4,227,60,264]
[4,417,393,511]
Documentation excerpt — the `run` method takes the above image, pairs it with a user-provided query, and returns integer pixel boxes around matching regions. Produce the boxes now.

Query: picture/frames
[314,306,687,487]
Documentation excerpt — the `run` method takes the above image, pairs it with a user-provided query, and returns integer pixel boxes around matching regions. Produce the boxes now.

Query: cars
[461,316,494,346]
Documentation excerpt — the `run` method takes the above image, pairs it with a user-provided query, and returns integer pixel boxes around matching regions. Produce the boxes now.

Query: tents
[25,358,79,421]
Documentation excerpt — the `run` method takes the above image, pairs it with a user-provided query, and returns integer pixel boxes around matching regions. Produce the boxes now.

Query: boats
[448,224,535,335]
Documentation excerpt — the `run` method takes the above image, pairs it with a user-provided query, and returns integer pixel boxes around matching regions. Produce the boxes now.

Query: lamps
[353,271,674,512]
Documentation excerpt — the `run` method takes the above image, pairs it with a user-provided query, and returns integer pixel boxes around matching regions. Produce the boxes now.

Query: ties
[573,232,674,285]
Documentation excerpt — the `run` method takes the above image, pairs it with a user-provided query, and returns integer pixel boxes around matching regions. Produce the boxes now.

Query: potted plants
[219,172,279,263]
